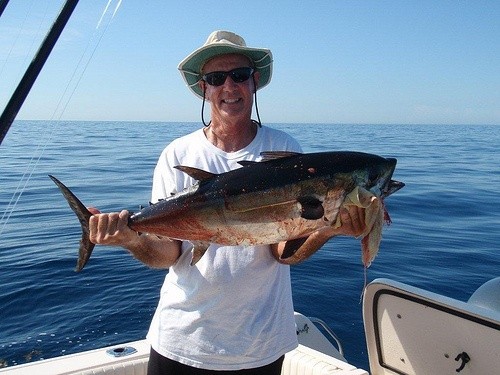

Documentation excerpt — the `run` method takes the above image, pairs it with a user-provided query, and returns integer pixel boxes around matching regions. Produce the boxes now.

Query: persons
[86,30,384,375]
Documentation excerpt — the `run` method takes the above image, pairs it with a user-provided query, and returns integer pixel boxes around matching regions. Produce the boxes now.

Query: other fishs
[45,150,405,273]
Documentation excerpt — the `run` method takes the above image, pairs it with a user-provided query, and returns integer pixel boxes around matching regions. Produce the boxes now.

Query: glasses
[201,67,255,87]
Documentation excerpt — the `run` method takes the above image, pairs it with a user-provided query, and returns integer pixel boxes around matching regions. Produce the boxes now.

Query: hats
[178,30,274,101]
